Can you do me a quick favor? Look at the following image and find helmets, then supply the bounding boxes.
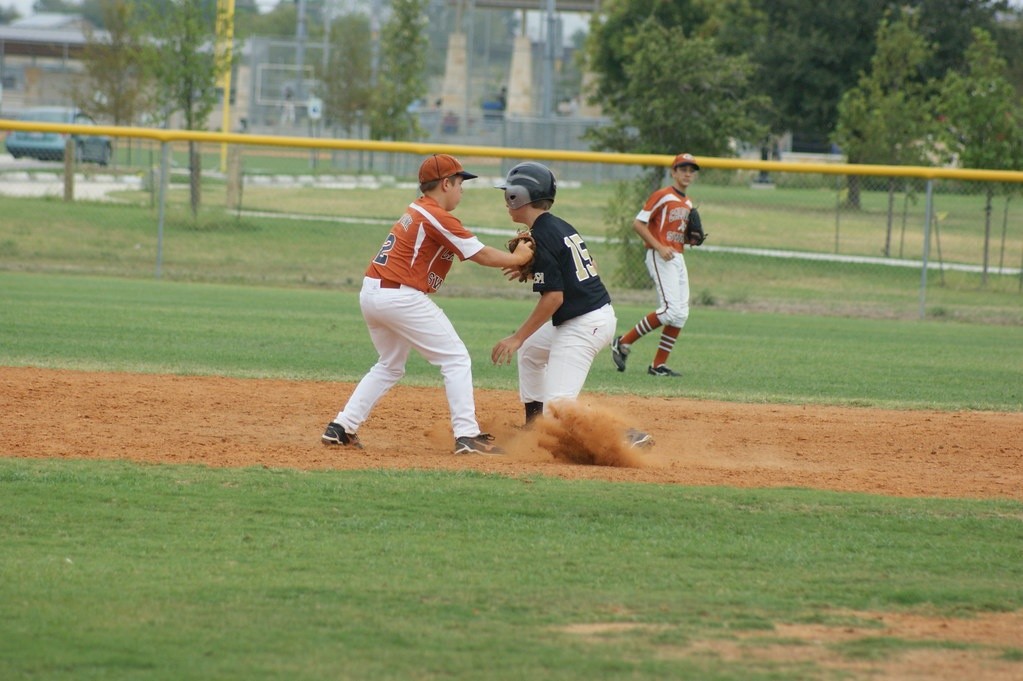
[494,162,557,210]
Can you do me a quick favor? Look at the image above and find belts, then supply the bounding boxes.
[380,279,400,289]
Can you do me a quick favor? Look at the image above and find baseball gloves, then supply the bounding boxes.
[684,207,704,246]
[504,226,536,283]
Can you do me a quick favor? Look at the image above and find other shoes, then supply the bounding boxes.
[626,428,655,450]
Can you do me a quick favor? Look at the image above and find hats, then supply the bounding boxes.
[419,153,478,183]
[673,153,700,171]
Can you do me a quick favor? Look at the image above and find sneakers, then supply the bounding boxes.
[455,434,506,456]
[648,363,682,377]
[323,422,364,449]
[611,335,631,372]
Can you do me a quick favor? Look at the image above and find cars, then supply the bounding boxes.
[7,108,112,166]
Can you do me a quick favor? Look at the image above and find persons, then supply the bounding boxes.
[492,160,656,454]
[610,154,700,376]
[321,154,534,458]
